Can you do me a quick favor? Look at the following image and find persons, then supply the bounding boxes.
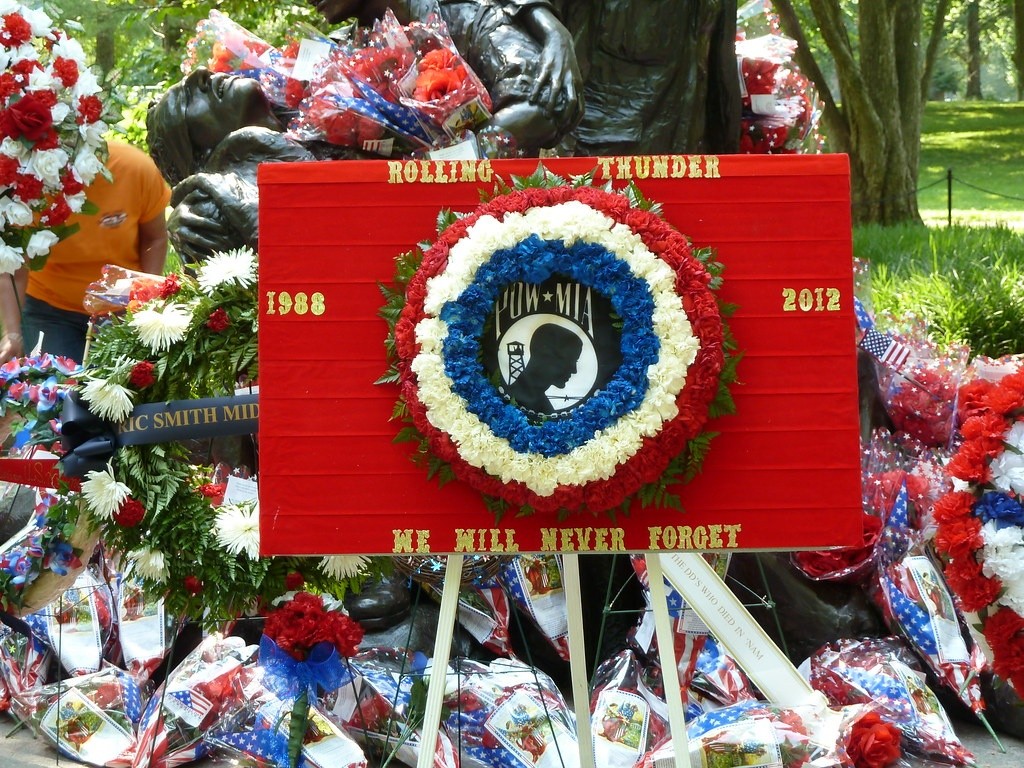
[0,141,170,368]
[144,2,752,282]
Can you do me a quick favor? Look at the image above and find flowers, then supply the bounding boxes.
[373,158,749,524]
[0,244,1024,768]
[734,0,830,159]
[0,0,125,278]
[168,0,527,160]
